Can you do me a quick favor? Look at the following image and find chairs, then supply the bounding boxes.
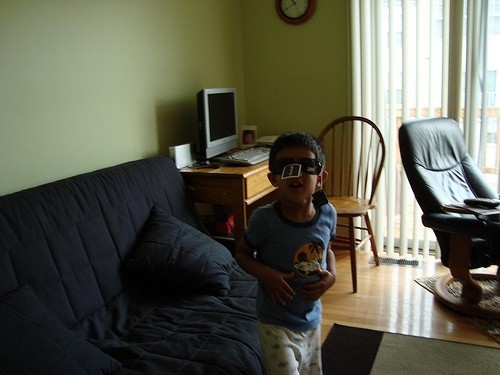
[398,117,500,318]
[315,116,385,294]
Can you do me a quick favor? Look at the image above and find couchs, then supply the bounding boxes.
[0,156,262,375]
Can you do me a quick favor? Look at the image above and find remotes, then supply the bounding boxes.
[464,198,500,209]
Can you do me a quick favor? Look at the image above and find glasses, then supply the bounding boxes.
[270,156,323,174]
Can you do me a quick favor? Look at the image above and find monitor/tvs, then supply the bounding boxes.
[197,87,239,158]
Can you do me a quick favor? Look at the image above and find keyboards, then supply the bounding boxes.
[220,146,271,164]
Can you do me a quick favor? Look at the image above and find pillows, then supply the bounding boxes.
[0,281,124,375]
[117,206,233,298]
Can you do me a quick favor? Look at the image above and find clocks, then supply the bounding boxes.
[274,0,318,26]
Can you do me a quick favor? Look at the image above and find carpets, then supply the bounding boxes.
[321,323,500,375]
[413,272,500,342]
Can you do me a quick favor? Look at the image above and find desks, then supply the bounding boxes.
[178,145,278,252]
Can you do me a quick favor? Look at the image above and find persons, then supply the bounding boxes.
[236,131,337,375]
